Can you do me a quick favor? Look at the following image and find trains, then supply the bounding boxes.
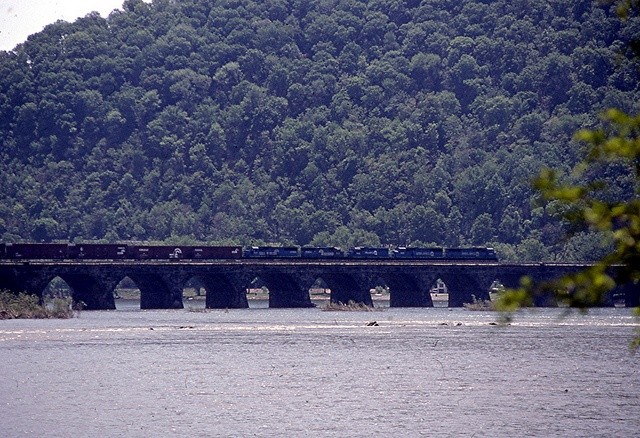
[0,243,503,261]
